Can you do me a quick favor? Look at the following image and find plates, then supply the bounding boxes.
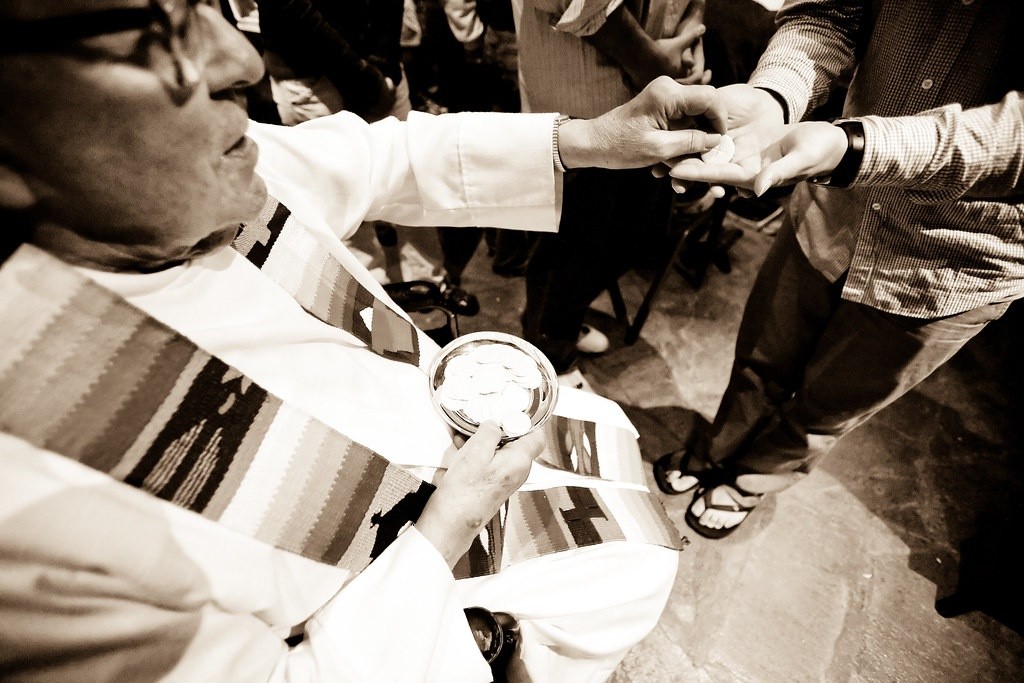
[427,330,560,445]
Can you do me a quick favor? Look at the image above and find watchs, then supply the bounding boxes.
[551,113,571,173]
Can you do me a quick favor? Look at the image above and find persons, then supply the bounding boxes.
[1,0,730,683]
[215,0,1024,541]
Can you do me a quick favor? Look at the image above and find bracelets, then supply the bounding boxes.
[814,121,864,187]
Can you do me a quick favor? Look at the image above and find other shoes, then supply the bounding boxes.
[575,324,610,353]
[556,369,594,394]
[436,283,481,318]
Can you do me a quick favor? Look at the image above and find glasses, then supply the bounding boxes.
[41,0,208,89]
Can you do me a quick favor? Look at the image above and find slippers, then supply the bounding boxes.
[685,484,758,540]
[654,448,708,495]
